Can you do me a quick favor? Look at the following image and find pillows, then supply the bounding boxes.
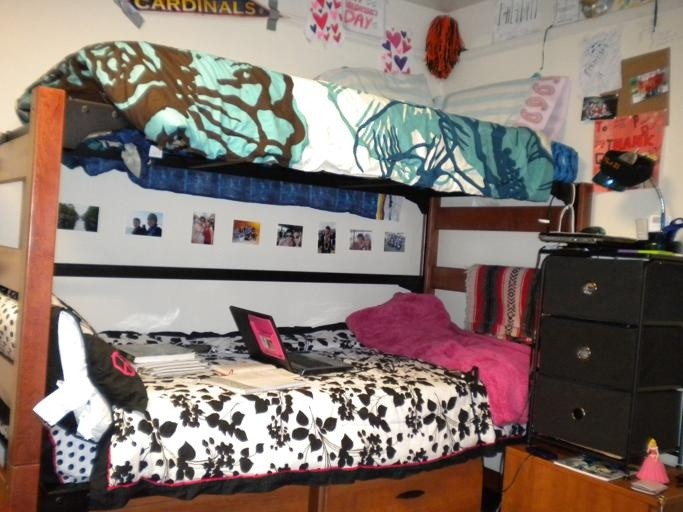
[315,67,567,131]
[0,290,149,446]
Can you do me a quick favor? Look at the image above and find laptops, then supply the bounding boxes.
[227,304,353,375]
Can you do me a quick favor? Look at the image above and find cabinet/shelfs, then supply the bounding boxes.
[497,254,681,511]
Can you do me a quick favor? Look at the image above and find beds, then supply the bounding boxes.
[0,41,593,512]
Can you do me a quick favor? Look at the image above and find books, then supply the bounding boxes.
[206,359,307,395]
[113,343,208,377]
[553,455,639,482]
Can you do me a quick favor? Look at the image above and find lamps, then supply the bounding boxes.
[591,149,673,244]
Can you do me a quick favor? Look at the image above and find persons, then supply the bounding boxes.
[320,225,334,252]
[278,229,301,247]
[636,438,671,484]
[636,68,665,99]
[357,233,370,250]
[583,101,608,118]
[192,216,213,244]
[131,213,161,237]
